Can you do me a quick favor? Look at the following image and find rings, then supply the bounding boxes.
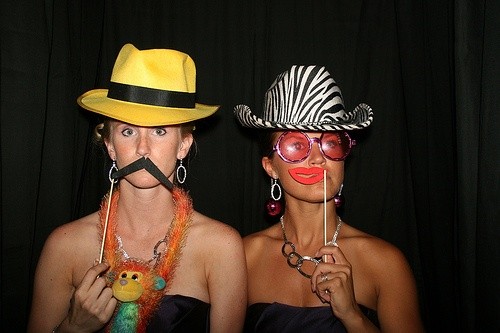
[321,273,328,282]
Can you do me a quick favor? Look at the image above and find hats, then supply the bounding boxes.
[78,45,220,126]
[233,65,374,129]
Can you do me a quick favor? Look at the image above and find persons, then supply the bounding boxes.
[235,65,421,333]
[30,43,248,333]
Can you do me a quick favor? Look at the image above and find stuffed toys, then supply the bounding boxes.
[98,187,193,332]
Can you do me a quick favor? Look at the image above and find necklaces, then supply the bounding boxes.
[280,215,342,278]
[114,231,169,270]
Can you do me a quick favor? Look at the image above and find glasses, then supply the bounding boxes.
[267,131,357,162]
